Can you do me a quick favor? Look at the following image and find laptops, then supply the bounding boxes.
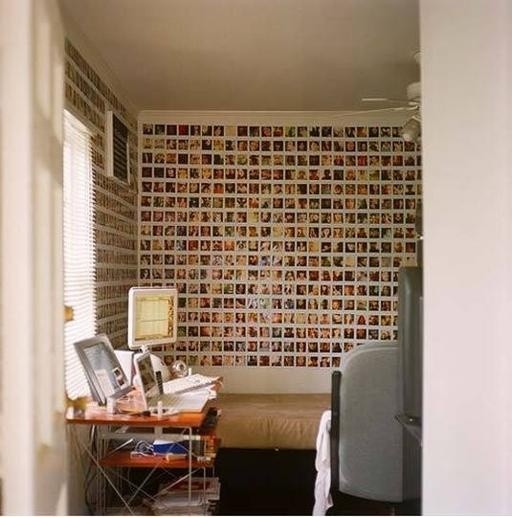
[134,352,211,412]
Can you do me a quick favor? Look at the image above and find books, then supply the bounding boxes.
[145,373,223,412]
[151,477,221,516]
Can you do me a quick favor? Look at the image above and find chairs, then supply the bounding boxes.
[330,343,406,516]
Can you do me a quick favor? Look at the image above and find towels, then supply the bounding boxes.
[312,411,334,516]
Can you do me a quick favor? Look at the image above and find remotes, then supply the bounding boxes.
[155,371,163,394]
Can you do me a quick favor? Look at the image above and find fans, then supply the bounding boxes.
[347,80,421,115]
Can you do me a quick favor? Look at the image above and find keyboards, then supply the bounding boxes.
[161,373,216,395]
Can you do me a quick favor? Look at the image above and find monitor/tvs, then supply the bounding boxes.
[127,287,178,348]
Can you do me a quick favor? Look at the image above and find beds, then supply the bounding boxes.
[111,353,333,450]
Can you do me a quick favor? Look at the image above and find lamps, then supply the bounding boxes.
[399,118,422,143]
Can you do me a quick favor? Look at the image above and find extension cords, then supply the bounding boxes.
[130,451,141,460]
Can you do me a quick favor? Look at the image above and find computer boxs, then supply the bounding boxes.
[113,407,221,505]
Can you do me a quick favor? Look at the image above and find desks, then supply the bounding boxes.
[66,373,218,517]
